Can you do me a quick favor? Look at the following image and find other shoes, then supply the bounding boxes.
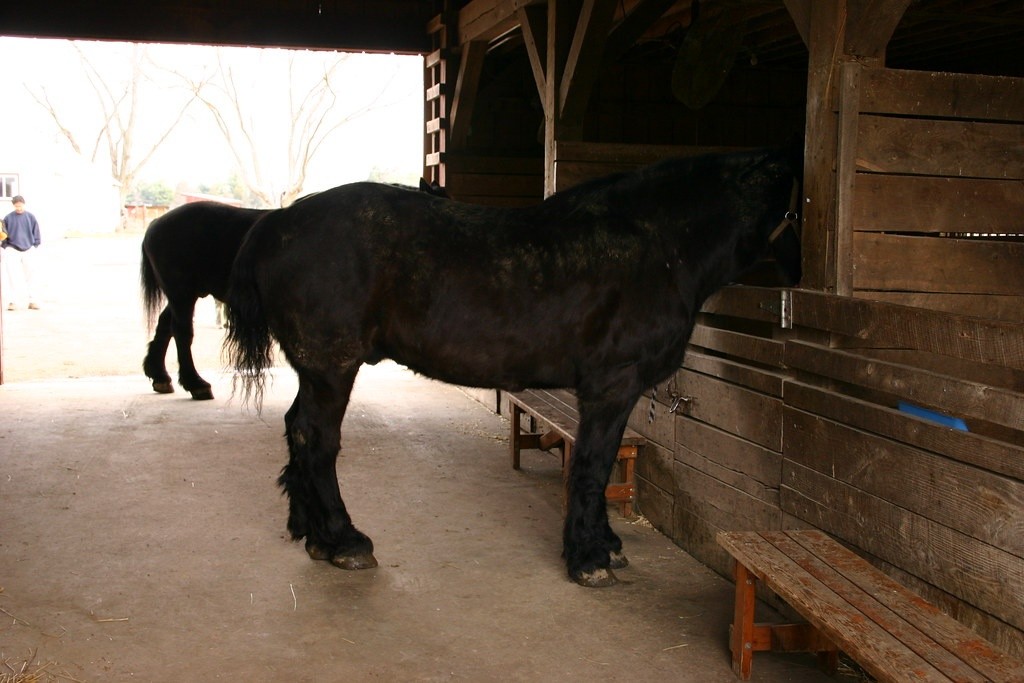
[29,303,39,310]
[8,303,15,311]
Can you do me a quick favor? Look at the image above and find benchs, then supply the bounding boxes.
[502,386,645,519]
[711,528,1023,683]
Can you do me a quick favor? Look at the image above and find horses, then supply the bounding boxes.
[225,144,803,588]
[142,202,277,401]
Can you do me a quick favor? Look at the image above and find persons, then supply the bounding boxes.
[1,195,45,311]
